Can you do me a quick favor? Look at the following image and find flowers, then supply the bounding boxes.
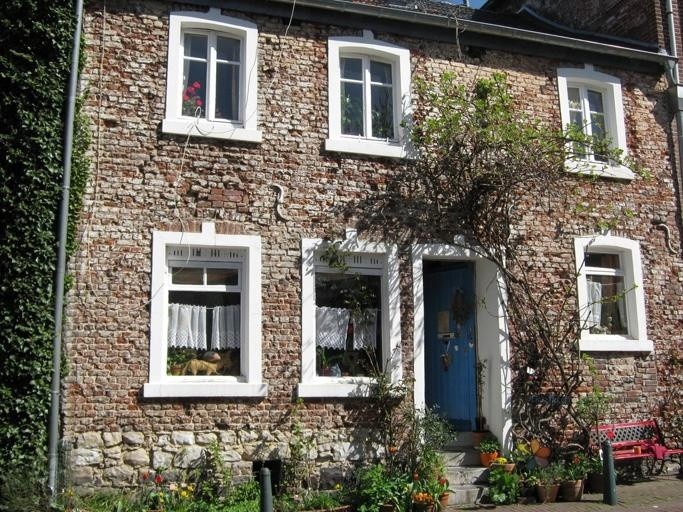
[183,80,205,117]
[403,468,456,512]
[495,456,507,465]
[567,450,591,479]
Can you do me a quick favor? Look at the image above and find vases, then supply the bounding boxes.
[561,478,588,502]
[491,462,515,474]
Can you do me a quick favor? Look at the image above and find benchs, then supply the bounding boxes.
[585,417,681,485]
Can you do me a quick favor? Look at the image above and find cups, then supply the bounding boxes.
[633,445,642,455]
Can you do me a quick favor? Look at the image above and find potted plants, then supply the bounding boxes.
[477,440,501,467]
[315,344,342,375]
[167,348,197,376]
[376,477,402,512]
[524,463,565,502]
